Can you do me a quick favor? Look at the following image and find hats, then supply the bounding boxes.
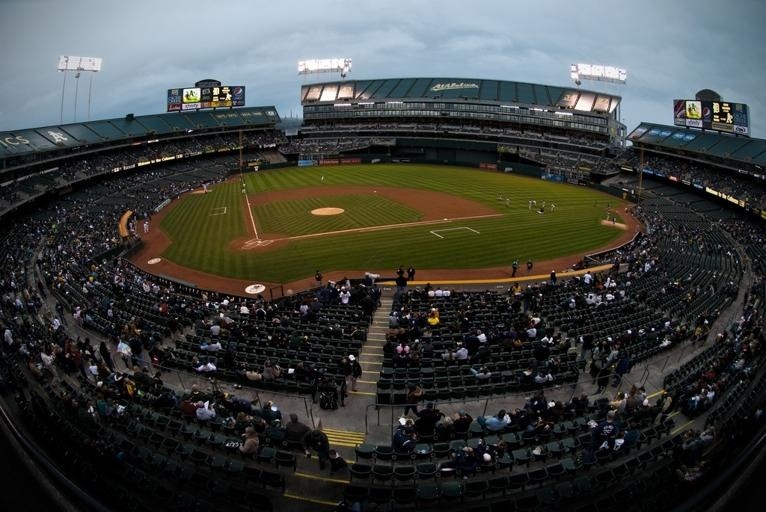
[348,354,356,361]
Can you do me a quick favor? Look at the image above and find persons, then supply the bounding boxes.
[494,191,560,216]
[621,145,765,512]
[1,120,241,512]
[242,244,621,511]
[604,203,617,226]
[242,124,621,185]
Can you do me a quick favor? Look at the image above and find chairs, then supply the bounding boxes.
[660,300,766,512]
[297,114,619,188]
[1,125,296,376]
[372,286,583,410]
[338,394,706,511]
[1,252,381,511]
[149,274,390,411]
[597,139,764,327]
[521,273,693,370]
